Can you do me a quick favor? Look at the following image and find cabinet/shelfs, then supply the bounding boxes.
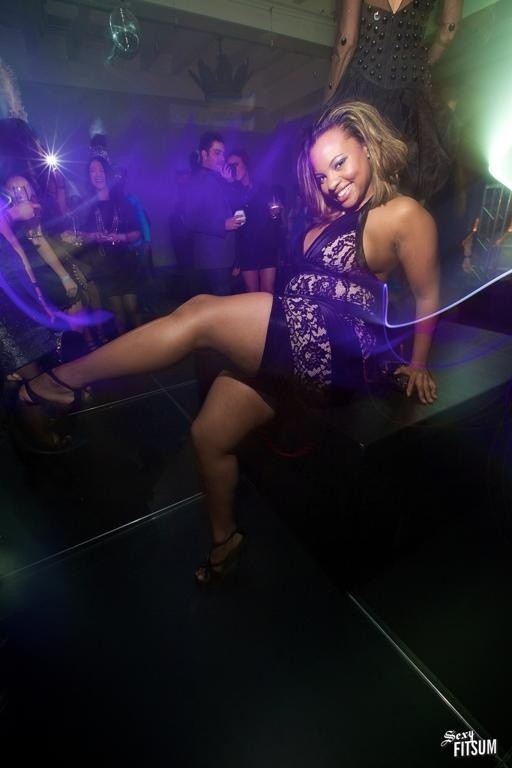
[191,322,512,598]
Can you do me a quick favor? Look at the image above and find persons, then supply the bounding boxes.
[7,102,441,581]
[320,0,464,112]
[1,119,309,378]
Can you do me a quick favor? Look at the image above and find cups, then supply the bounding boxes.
[234,210,245,225]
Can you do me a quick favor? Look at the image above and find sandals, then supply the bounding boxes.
[193,527,253,587]
[85,333,107,351]
[1,369,76,410]
